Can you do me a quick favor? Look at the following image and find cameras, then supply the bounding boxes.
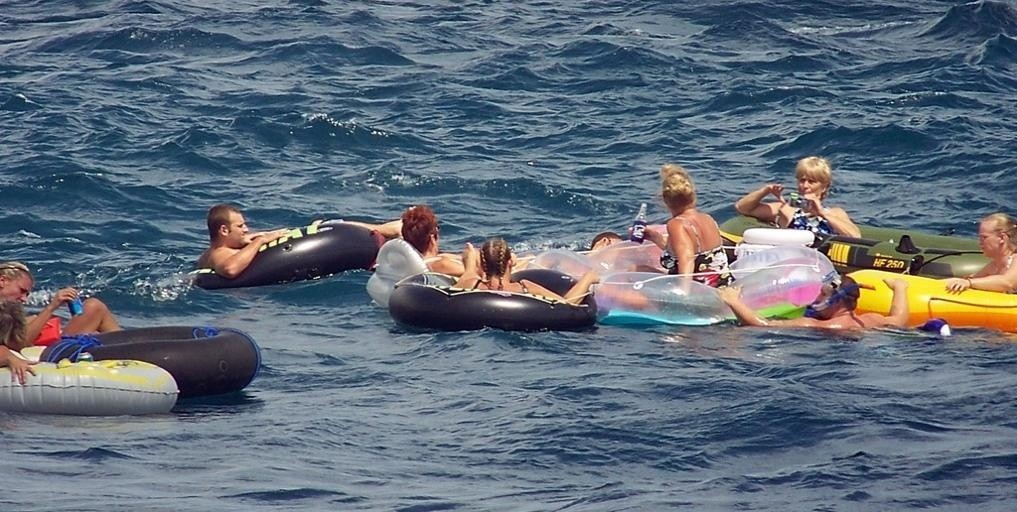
[788,193,809,208]
[65,291,90,315]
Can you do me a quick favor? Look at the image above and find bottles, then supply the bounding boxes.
[629,204,649,243]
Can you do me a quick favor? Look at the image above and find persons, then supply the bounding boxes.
[630,161,731,289]
[946,213,1017,293]
[1,259,118,339]
[199,205,419,278]
[402,204,537,275]
[0,300,36,385]
[717,271,910,329]
[591,232,626,253]
[735,156,862,239]
[451,237,601,305]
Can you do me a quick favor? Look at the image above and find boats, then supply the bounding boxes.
[719,213,1016,332]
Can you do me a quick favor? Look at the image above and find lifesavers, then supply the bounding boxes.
[389,268,598,333]
[845,269,1017,333]
[365,238,429,309]
[35,325,262,399]
[0,346,180,416]
[194,223,379,290]
[526,239,836,326]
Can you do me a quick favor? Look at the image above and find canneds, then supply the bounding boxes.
[75,352,93,363]
[67,298,85,318]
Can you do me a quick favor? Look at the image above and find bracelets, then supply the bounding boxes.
[968,278,975,289]
